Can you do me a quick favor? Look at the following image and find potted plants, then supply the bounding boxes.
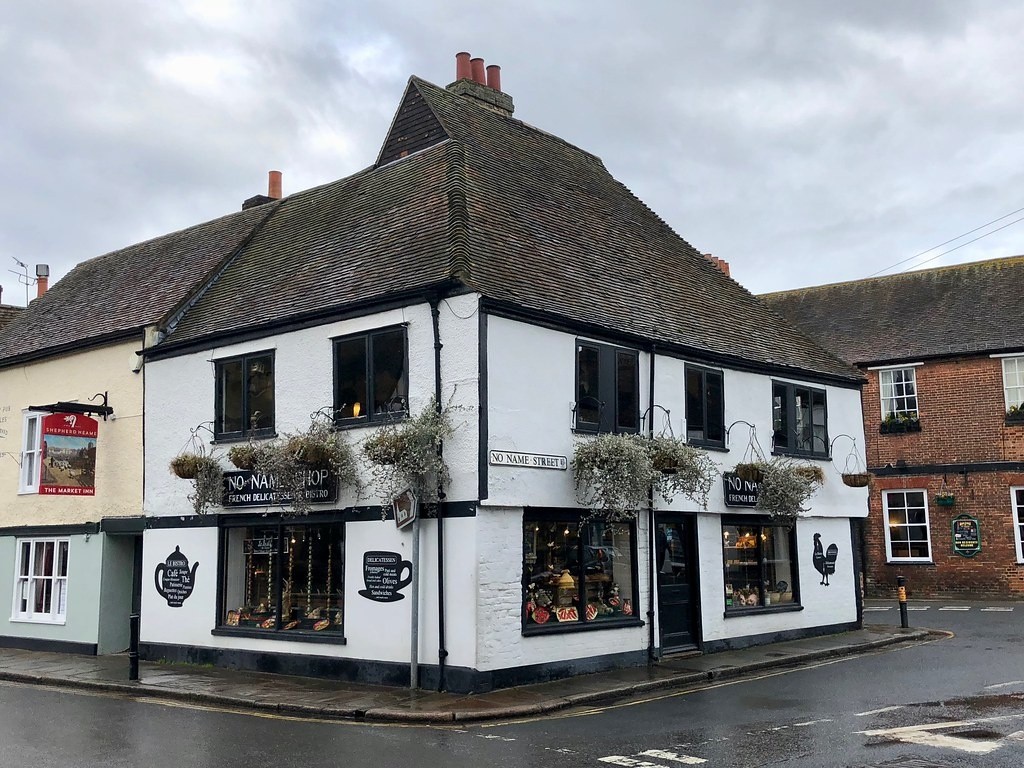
[933,492,957,507]
[226,384,875,523]
[168,444,230,515]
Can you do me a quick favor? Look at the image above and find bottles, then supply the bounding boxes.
[726,594,733,607]
[765,594,770,604]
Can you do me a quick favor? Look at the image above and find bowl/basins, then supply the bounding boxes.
[783,591,792,602]
[770,593,780,604]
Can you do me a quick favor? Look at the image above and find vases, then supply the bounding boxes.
[1007,414,1015,420]
[1014,413,1024,420]
[882,421,919,431]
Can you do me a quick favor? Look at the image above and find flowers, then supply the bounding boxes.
[881,405,1024,429]
